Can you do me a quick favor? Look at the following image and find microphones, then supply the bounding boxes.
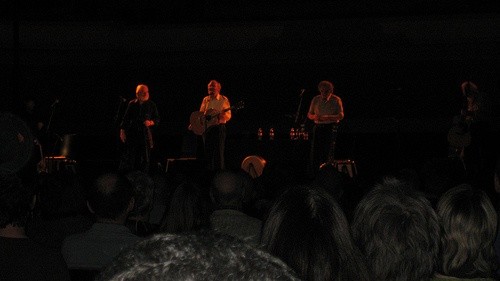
[299,89,305,96]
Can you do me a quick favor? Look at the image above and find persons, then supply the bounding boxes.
[456,82,482,163]
[189,80,232,171]
[307,81,344,156]
[120,83,160,168]
[0,155,500,281]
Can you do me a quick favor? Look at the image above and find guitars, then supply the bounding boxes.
[188,103,244,135]
[35,140,52,175]
[445,102,478,153]
